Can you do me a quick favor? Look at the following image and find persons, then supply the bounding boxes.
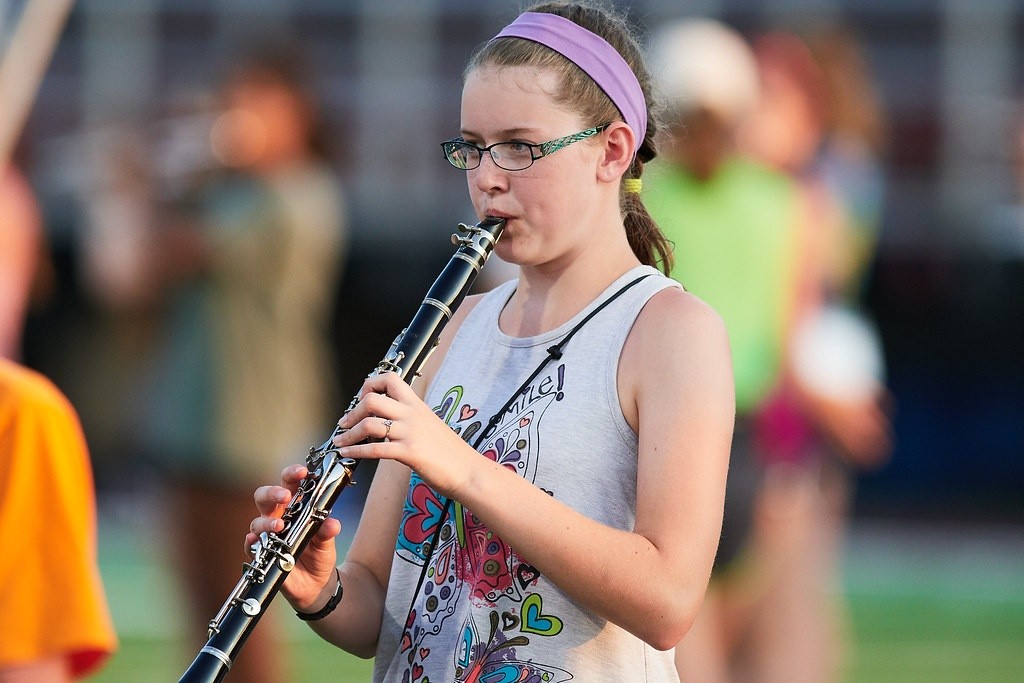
[627,18,896,679]
[0,161,120,681]
[242,3,737,683]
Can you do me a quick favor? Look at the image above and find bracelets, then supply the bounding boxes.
[295,567,344,622]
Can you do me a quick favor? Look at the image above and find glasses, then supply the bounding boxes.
[440,121,613,171]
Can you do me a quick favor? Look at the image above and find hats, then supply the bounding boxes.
[643,17,756,124]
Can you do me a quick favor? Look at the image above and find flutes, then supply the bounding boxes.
[175,211,513,683]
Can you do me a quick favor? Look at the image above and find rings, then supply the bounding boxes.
[382,419,393,440]
